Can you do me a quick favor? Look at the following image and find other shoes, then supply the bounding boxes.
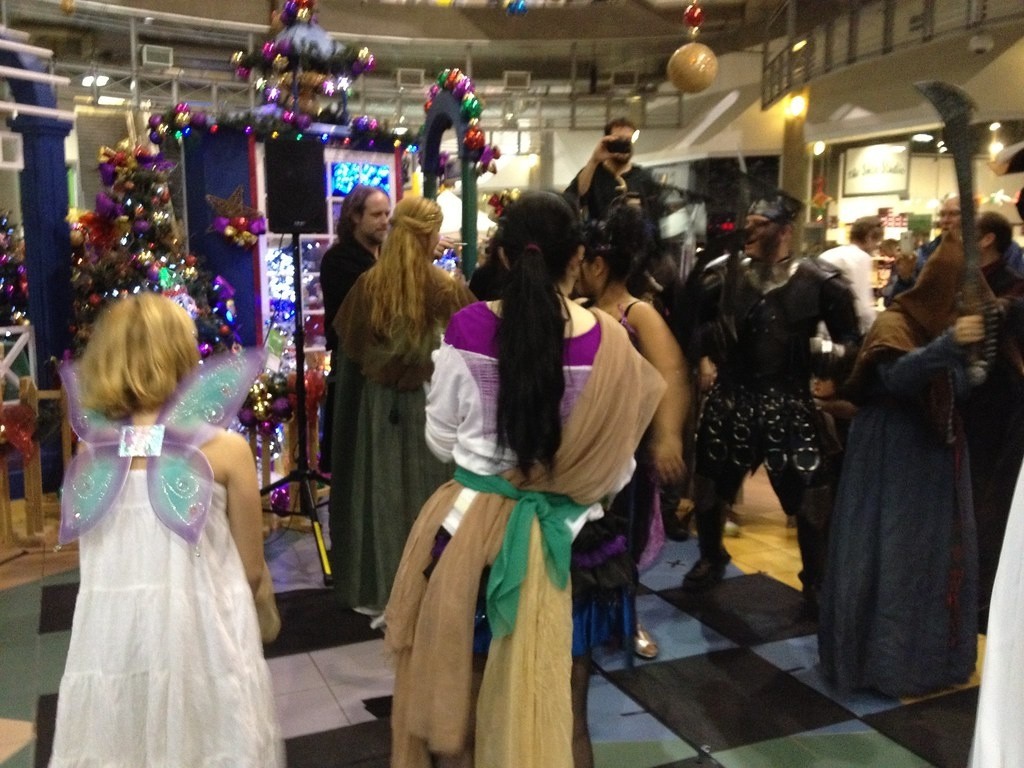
[633,624,657,657]
[684,547,730,591]
[802,585,819,604]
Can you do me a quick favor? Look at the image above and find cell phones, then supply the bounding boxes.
[606,139,634,154]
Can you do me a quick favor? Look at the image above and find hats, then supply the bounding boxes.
[748,198,792,226]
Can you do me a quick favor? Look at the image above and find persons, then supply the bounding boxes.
[683,190,864,622]
[567,218,690,663]
[48,292,287,768]
[817,238,1000,698]
[629,184,1024,498]
[562,118,660,225]
[322,182,393,587]
[384,189,666,768]
[336,191,474,625]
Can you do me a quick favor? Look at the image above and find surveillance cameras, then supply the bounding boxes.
[967,29,993,56]
